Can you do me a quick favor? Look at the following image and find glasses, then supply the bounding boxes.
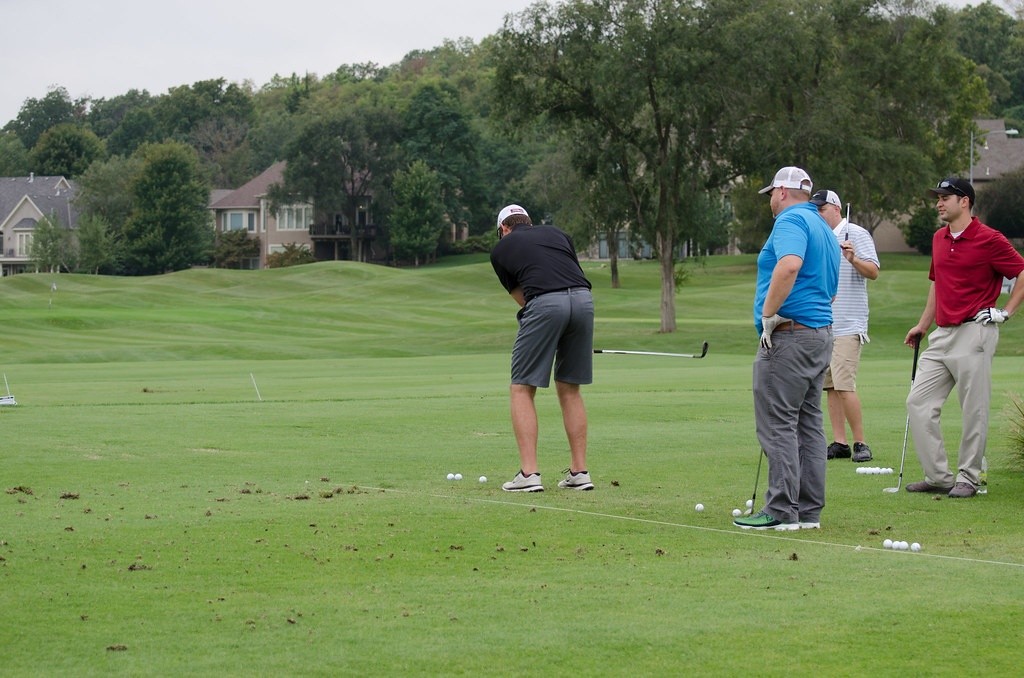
[935,180,967,195]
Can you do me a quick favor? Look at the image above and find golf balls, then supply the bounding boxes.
[447,473,454,480]
[856,467,893,474]
[695,504,704,512]
[746,500,752,508]
[455,474,462,480]
[911,543,920,551]
[732,508,741,517]
[479,476,487,483]
[899,541,908,550]
[892,541,900,549]
[883,539,892,548]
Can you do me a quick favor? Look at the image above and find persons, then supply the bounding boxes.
[810,190,880,463]
[903,178,1024,497]
[490,205,594,494]
[733,167,841,529]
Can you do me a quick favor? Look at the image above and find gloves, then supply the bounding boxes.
[759,313,792,349]
[972,307,1006,327]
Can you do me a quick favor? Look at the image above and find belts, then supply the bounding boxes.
[774,320,813,331]
[542,285,592,293]
[961,318,976,323]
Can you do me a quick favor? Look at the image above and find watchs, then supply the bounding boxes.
[998,307,1009,322]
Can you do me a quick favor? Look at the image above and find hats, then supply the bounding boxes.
[925,176,978,204]
[496,204,533,237]
[758,164,815,195]
[810,189,844,210]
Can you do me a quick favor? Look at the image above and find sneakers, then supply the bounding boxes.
[797,518,821,529]
[556,467,595,490]
[500,469,544,492]
[731,510,800,532]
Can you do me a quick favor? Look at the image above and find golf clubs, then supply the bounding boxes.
[882,333,922,493]
[743,448,763,517]
[591,341,708,358]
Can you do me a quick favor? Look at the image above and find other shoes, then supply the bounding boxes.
[851,442,874,462]
[824,440,852,460]
[906,478,955,495]
[948,480,976,499]
